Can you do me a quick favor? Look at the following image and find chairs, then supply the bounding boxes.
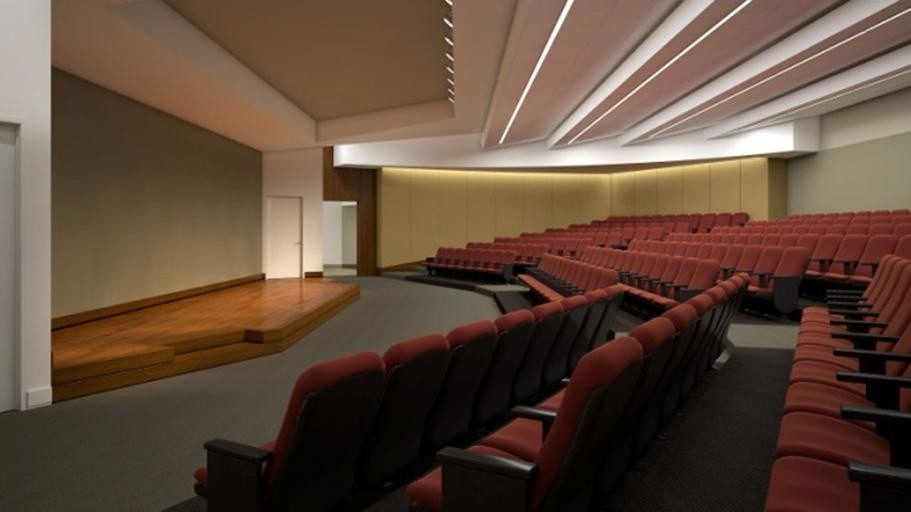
[197,255,911,510]
[422,213,910,316]
